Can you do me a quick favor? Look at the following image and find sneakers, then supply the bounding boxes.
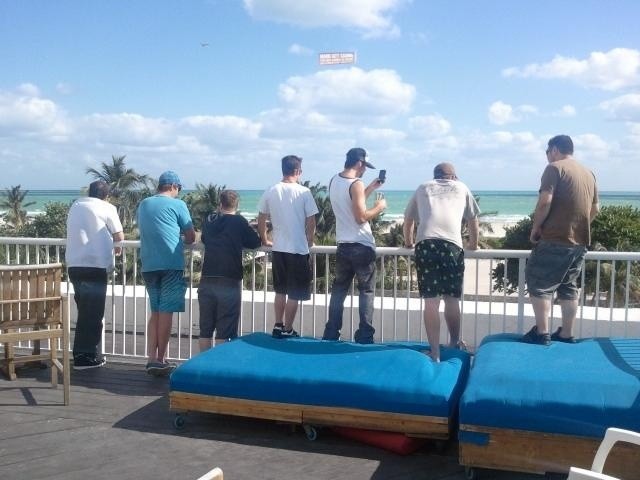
[272,323,299,339]
[73,356,107,369]
[551,326,576,343]
[518,325,551,346]
[146,360,176,376]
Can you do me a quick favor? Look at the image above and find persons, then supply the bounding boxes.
[257,155,318,339]
[136,170,197,375]
[197,190,261,352]
[321,146,389,343]
[65,181,124,371]
[403,161,480,363]
[518,135,599,345]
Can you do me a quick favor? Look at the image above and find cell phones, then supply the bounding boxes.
[379,170,386,183]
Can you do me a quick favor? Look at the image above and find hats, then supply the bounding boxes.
[159,171,181,185]
[346,148,376,169]
[434,162,458,179]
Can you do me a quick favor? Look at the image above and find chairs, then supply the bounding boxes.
[563,425,640,480]
[1,261,73,408]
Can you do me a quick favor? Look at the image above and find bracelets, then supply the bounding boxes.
[405,244,413,248]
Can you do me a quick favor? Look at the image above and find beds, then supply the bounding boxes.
[454,332,640,479]
[165,330,472,443]
